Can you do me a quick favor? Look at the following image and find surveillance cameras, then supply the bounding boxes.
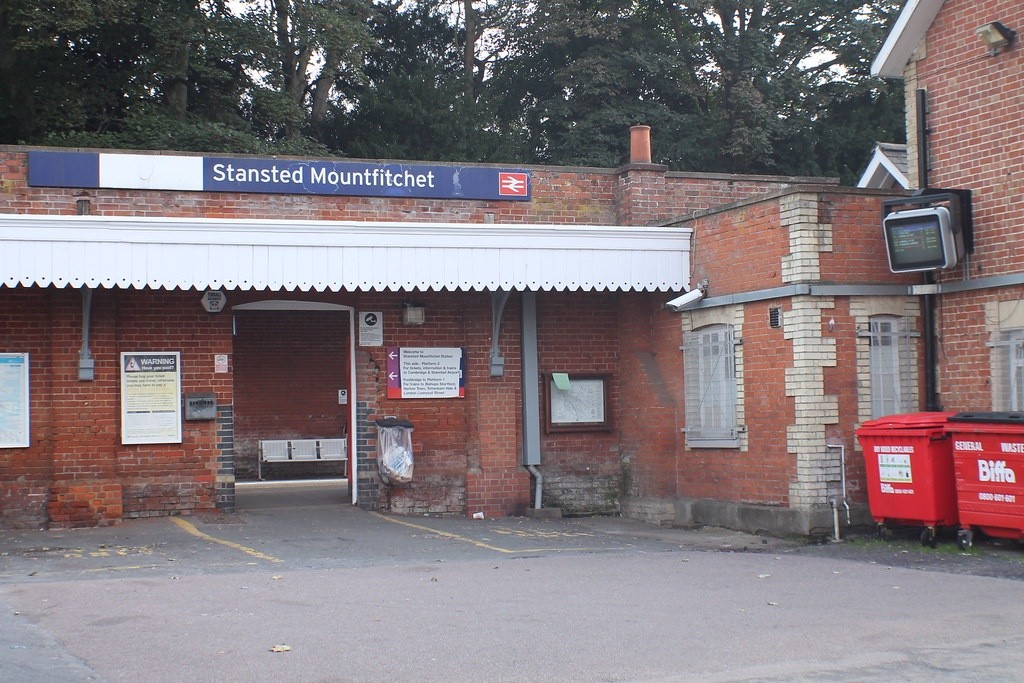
[666,289,703,311]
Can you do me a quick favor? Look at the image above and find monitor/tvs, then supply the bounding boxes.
[883,206,965,274]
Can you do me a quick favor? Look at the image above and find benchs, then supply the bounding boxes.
[256,441,348,479]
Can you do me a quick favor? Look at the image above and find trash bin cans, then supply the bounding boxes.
[944,410,1023,550]
[855,413,960,548]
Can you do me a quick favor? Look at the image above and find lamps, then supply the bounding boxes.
[975,21,1016,48]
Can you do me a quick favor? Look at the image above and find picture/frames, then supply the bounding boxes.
[542,372,612,434]
